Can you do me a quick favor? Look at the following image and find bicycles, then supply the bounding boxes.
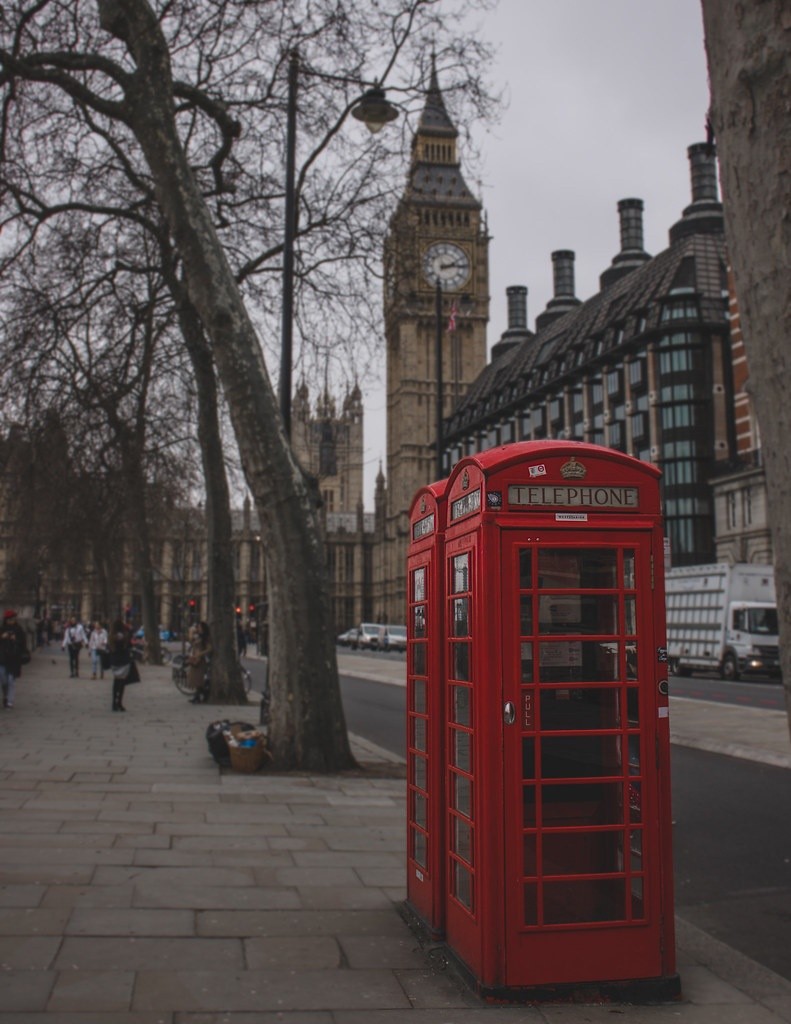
[176,661,252,698]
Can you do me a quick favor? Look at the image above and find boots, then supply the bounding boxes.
[188,680,210,705]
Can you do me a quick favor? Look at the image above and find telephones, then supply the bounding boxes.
[521,620,587,698]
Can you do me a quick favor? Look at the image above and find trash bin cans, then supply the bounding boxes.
[207,718,268,773]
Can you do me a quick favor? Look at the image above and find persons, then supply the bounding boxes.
[0,607,245,713]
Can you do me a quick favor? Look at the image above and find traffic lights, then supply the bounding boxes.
[237,607,242,617]
[249,604,255,613]
[190,597,195,613]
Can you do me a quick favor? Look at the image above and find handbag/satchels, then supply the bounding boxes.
[18,649,31,665]
[96,648,111,670]
[72,642,82,650]
[187,669,205,689]
[112,658,141,686]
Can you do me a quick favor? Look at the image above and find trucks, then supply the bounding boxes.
[664,564,782,680]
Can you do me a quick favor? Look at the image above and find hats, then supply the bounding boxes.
[2,610,18,620]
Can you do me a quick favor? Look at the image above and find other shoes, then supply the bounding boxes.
[113,701,126,711]
[90,672,97,680]
[5,701,13,707]
[100,672,105,680]
[70,670,79,678]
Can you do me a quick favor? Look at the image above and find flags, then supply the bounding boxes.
[446,302,459,333]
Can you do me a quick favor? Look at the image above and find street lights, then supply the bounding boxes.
[280,51,399,443]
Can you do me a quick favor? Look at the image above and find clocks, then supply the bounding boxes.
[420,241,470,290]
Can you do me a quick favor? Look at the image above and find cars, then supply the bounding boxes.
[384,624,408,650]
[359,623,387,649]
